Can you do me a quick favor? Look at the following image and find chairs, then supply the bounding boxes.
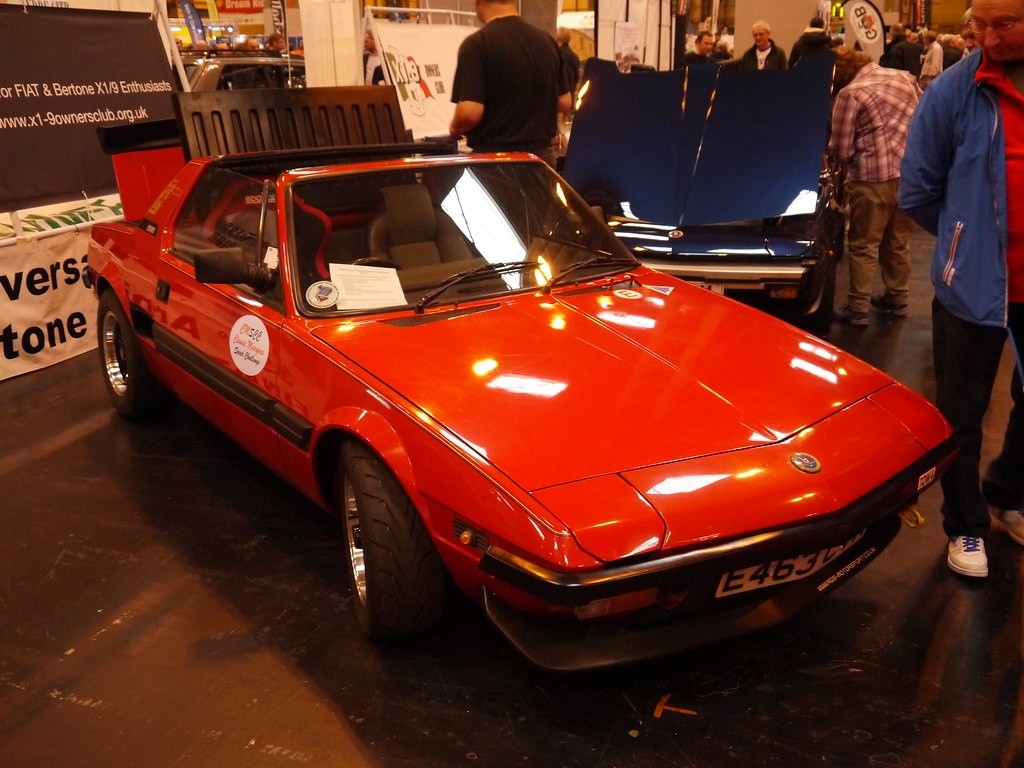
[367,183,475,270]
[199,172,332,283]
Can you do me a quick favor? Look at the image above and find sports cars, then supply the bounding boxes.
[86,134,968,676]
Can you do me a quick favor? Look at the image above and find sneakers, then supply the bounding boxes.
[842,305,870,326]
[988,500,1024,543]
[871,295,909,315]
[947,535,987,576]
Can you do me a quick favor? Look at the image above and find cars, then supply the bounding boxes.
[563,48,846,334]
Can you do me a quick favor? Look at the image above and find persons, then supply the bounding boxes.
[879,23,925,81]
[740,20,787,70]
[363,30,386,86]
[896,0,1024,577]
[192,40,211,58]
[682,31,716,70]
[834,36,844,46]
[714,30,722,46]
[960,7,978,53]
[787,16,836,70]
[232,38,261,89]
[918,30,943,91]
[710,40,731,60]
[828,50,924,325]
[943,34,963,71]
[449,0,572,245]
[905,25,918,46]
[174,37,191,56]
[262,32,289,88]
[556,27,580,111]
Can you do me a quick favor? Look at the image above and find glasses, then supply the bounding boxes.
[968,15,1024,34]
